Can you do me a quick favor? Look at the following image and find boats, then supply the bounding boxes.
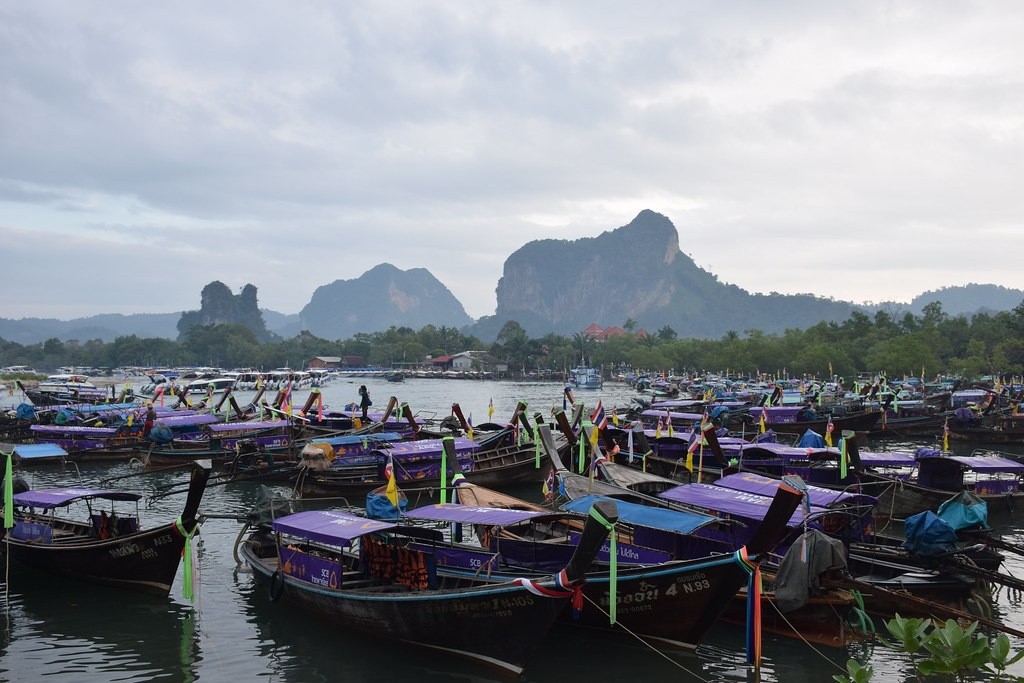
[0,442,213,597]
[0,365,1024,673]
